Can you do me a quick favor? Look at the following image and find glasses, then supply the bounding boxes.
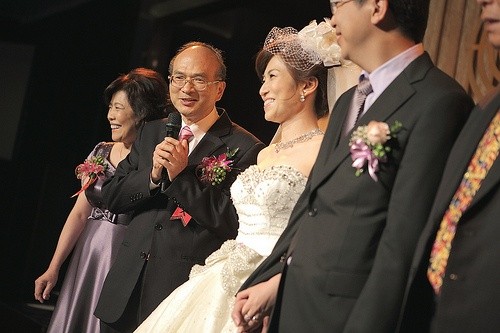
[330,0,348,15]
[167,73,221,90]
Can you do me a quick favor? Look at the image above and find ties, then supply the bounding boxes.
[176,126,195,155]
[425,112,500,293]
[338,76,373,147]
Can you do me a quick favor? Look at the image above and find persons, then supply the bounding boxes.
[84,41,267,333]
[232,0,476,333]
[131,17,367,333]
[394,0,500,333]
[34,67,178,333]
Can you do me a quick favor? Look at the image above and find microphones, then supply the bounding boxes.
[160,112,182,182]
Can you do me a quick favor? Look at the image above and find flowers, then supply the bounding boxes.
[195,145,243,188]
[348,119,405,184]
[298,15,353,68]
[74,155,111,181]
